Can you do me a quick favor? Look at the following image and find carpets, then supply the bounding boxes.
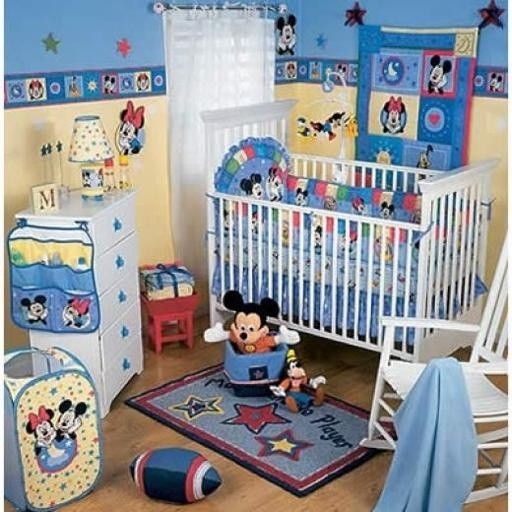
[125,364,395,501]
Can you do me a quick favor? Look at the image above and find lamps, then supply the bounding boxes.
[68,117,117,200]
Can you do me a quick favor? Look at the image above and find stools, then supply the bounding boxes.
[148,311,196,353]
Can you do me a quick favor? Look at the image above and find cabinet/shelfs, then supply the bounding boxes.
[15,187,145,417]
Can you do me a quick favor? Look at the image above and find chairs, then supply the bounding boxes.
[361,234,507,505]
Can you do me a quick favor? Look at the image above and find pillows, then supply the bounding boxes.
[215,136,290,217]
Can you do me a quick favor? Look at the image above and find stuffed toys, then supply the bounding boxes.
[202,291,301,354]
[296,80,358,141]
[270,348,326,412]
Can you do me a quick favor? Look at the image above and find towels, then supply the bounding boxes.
[374,347,480,511]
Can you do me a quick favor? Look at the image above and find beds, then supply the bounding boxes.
[199,104,499,366]
